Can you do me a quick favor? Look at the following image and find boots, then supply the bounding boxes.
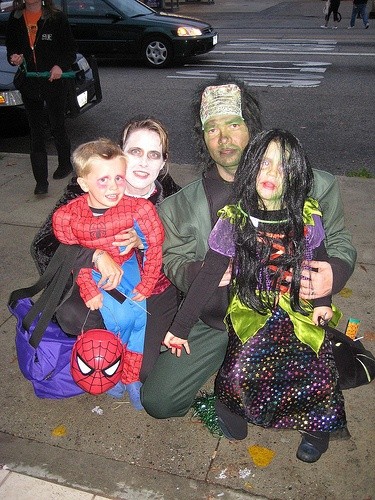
[30,149,50,194]
[52,138,73,179]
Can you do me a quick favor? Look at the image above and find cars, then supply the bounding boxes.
[0,0,219,70]
[0,44,104,138]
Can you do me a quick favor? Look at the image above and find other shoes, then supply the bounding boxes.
[211,396,250,440]
[364,23,369,29]
[332,26,338,30]
[348,26,355,30]
[296,437,329,464]
[320,25,327,28]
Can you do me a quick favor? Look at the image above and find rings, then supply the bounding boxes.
[310,289,314,295]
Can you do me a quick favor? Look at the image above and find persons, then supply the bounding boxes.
[321,0,341,29]
[5,0,78,195]
[349,0,370,29]
[31,73,357,464]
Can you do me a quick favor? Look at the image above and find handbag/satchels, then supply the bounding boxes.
[13,63,27,91]
[319,317,375,388]
[323,0,330,15]
[7,242,87,399]
